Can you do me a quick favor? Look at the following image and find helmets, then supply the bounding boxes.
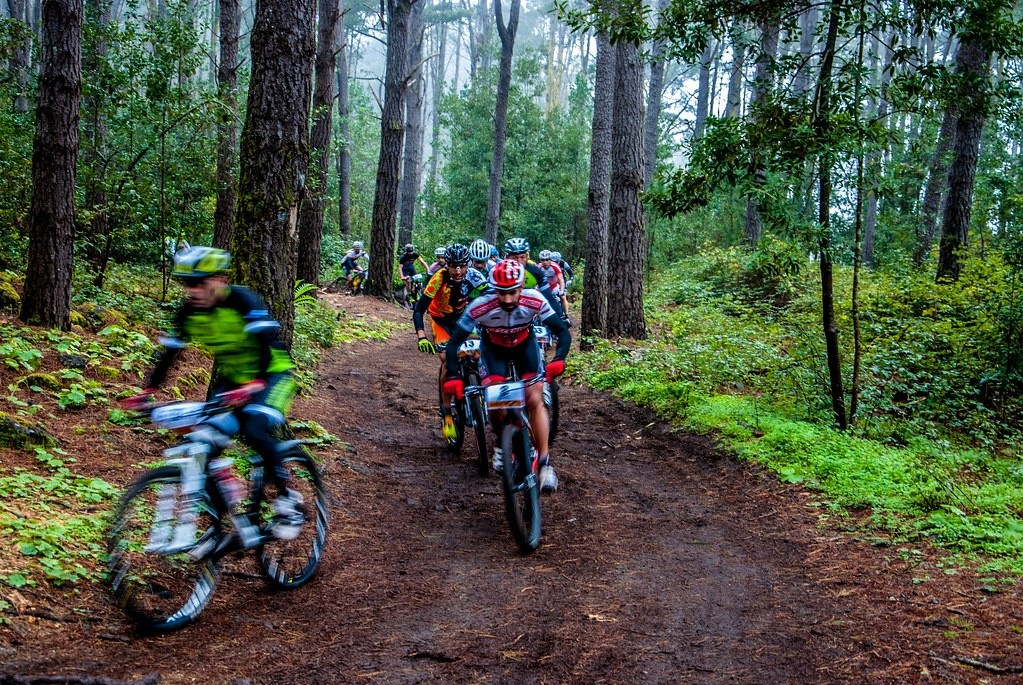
[434,247,446,256]
[444,244,470,262]
[170,247,231,274]
[552,252,562,261]
[488,259,524,291]
[354,241,363,250]
[405,244,414,251]
[505,238,530,253]
[539,250,552,258]
[490,245,499,256]
[469,239,491,262]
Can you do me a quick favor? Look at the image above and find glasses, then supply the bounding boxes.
[495,285,522,295]
[170,276,217,287]
[447,262,468,268]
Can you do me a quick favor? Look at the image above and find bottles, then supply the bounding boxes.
[209,457,245,504]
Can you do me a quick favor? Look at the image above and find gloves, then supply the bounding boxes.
[545,361,565,382]
[417,337,436,355]
[444,380,463,398]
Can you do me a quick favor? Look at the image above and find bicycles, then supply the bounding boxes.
[104,378,330,640]
[533,279,574,363]
[418,334,493,478]
[400,269,427,310]
[452,366,565,556]
[335,265,369,294]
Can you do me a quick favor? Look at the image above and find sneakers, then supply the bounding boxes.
[492,446,505,472]
[272,491,304,540]
[441,415,459,444]
[538,466,558,493]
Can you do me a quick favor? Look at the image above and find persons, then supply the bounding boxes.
[504,238,571,328]
[398,244,430,309]
[467,238,504,280]
[421,247,447,296]
[412,243,491,444]
[537,249,564,307]
[340,240,369,290]
[550,252,574,324]
[160,228,191,267]
[122,245,306,556]
[442,258,572,495]
